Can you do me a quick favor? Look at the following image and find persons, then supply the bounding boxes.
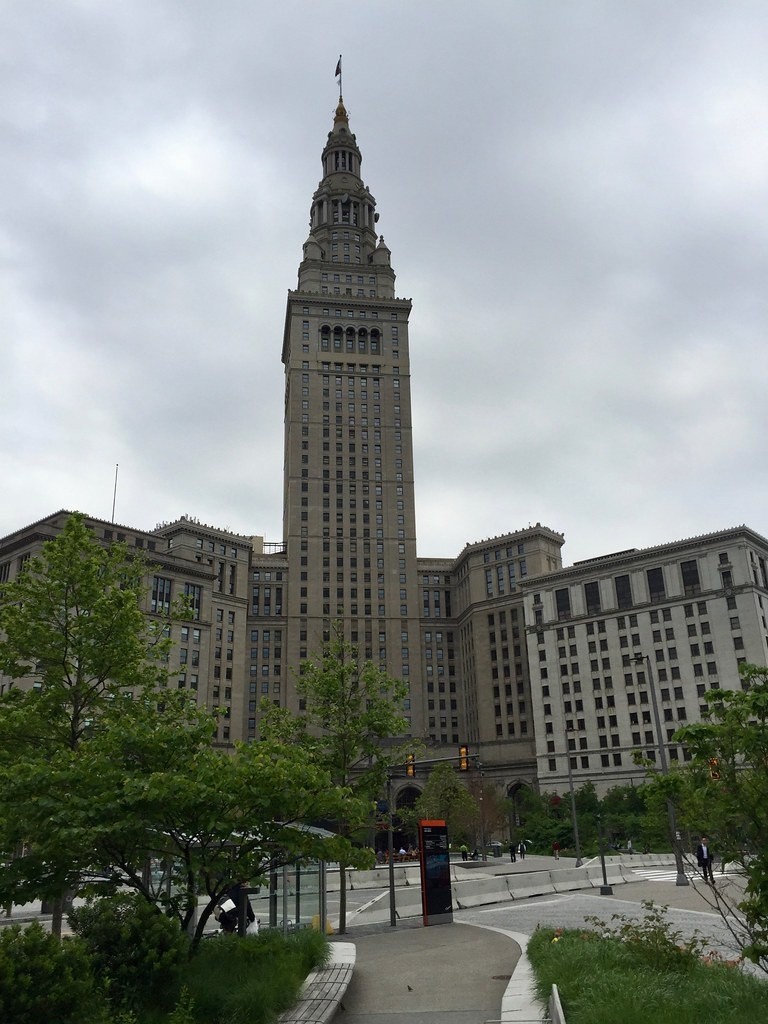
[697,837,715,884]
[628,838,633,855]
[408,843,413,852]
[509,842,516,863]
[399,847,407,861]
[377,848,396,864]
[414,847,419,853]
[493,846,502,857]
[223,881,259,934]
[460,843,467,861]
[467,849,478,860]
[518,840,526,859]
[643,843,650,854]
[608,841,621,850]
[552,840,560,860]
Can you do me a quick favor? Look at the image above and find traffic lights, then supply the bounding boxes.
[709,757,720,780]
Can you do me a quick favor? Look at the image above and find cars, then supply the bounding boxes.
[676,832,700,843]
[486,841,504,847]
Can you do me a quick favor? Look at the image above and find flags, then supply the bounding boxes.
[335,59,340,77]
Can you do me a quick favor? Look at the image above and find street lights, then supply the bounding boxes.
[564,728,584,867]
[626,655,689,886]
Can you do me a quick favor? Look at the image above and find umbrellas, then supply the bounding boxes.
[488,841,504,846]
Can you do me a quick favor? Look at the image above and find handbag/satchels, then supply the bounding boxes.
[246,918,258,936]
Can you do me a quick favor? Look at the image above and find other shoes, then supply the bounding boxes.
[712,881,715,885]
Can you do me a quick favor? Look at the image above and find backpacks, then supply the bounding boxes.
[212,895,238,926]
